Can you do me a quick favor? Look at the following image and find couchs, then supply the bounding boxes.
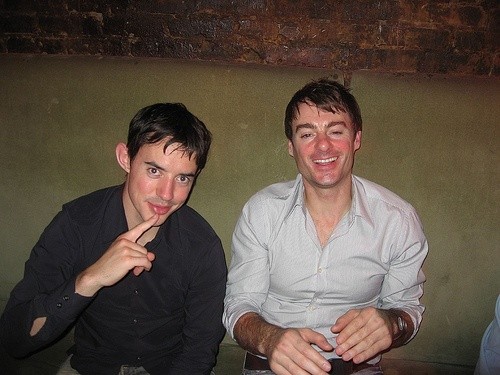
[0,52,500,375]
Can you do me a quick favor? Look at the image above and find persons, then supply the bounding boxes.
[0,103,228,375]
[222,79,429,375]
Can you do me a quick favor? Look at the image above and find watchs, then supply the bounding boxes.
[384,309,408,340]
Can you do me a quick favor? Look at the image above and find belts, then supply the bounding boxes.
[242,351,382,375]
